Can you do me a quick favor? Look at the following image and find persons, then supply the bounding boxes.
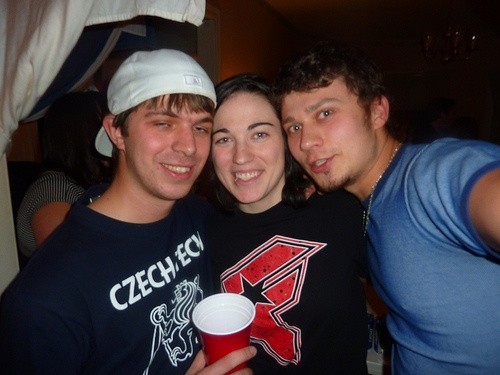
[0,49,257,375]
[280,46,500,374]
[210,72,388,375]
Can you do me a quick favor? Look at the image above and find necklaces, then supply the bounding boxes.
[363,141,403,236]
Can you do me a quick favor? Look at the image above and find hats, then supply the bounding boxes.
[95,49,217,158]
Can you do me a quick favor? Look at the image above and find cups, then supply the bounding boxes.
[192,293,256,375]
